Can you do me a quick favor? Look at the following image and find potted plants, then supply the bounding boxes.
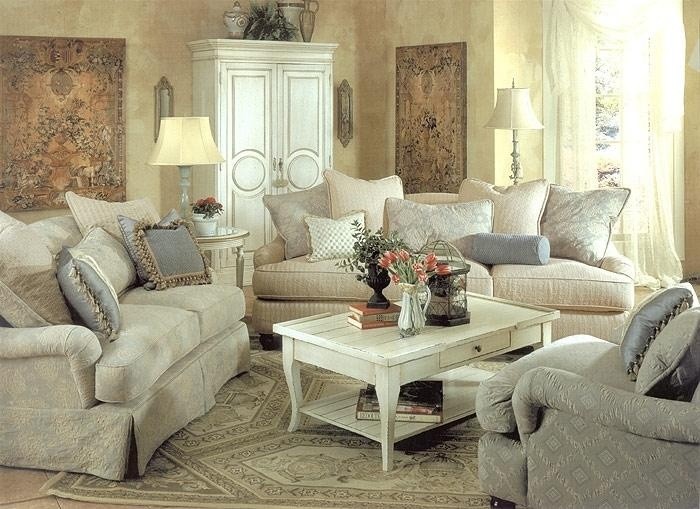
[333,215,412,310]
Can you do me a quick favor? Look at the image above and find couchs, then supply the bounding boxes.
[249,189,642,353]
[0,208,251,482]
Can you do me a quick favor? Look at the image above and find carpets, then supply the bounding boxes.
[37,328,538,509]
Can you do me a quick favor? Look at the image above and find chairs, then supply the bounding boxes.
[475,280,699,509]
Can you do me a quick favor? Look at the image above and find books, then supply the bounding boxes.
[366,386,441,416]
[350,311,402,323]
[355,388,444,425]
[347,315,399,329]
[348,302,402,317]
[364,379,445,410]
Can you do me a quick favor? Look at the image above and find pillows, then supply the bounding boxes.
[620,279,699,382]
[464,229,554,268]
[54,187,213,343]
[321,169,404,240]
[539,183,631,269]
[300,209,368,262]
[457,177,551,237]
[262,182,329,260]
[385,196,495,253]
[634,307,699,404]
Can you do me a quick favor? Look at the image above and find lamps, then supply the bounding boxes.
[482,78,546,184]
[147,115,225,221]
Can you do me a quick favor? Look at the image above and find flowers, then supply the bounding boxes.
[190,197,227,219]
[373,247,454,291]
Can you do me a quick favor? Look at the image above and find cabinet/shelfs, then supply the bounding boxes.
[186,38,342,292]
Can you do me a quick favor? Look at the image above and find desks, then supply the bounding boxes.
[184,220,250,290]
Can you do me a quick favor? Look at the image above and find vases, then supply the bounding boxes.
[192,211,219,236]
[297,0,320,43]
[396,282,432,337]
[277,0,306,42]
[223,1,252,39]
